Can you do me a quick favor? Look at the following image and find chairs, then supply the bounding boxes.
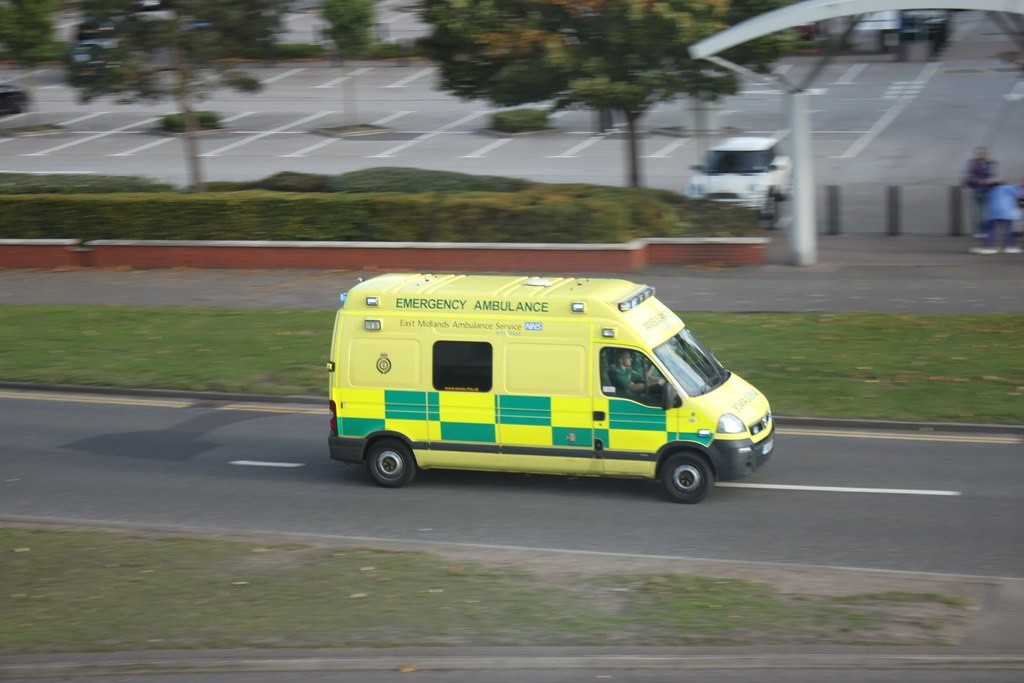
[601,350,616,396]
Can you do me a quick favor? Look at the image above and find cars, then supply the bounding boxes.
[900,9,950,50]
[72,38,124,84]
[686,137,792,222]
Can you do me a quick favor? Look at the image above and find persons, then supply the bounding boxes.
[969,182,1024,255]
[609,349,666,394]
[963,146,1002,238]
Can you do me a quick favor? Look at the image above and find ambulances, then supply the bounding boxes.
[325,271,775,505]
